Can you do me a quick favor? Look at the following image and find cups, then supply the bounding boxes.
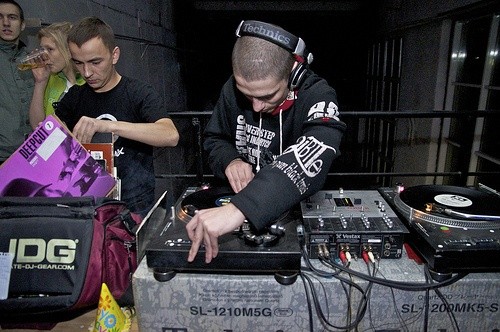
[17,47,50,71]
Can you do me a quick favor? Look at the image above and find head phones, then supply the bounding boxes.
[236,20,315,91]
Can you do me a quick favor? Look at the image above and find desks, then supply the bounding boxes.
[132,243,500,332]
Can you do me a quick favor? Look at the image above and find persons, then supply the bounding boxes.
[26,22,86,129]
[53,16,180,306]
[184,19,347,265]
[0,0,36,166]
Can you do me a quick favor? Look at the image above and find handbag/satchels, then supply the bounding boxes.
[0,197,144,317]
